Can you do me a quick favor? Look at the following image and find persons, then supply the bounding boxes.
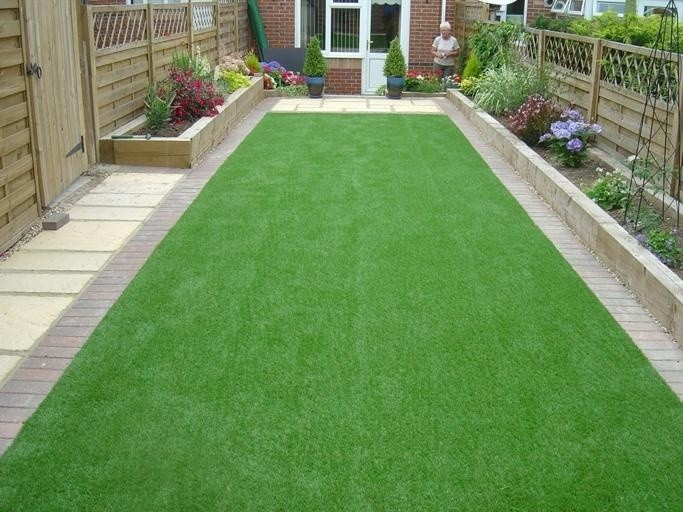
[429,21,461,79]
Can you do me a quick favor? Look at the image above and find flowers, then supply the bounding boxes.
[259,60,304,91]
[509,95,603,167]
[155,69,224,127]
[405,70,461,93]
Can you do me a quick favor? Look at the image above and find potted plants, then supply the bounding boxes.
[382,33,406,99]
[301,34,326,98]
